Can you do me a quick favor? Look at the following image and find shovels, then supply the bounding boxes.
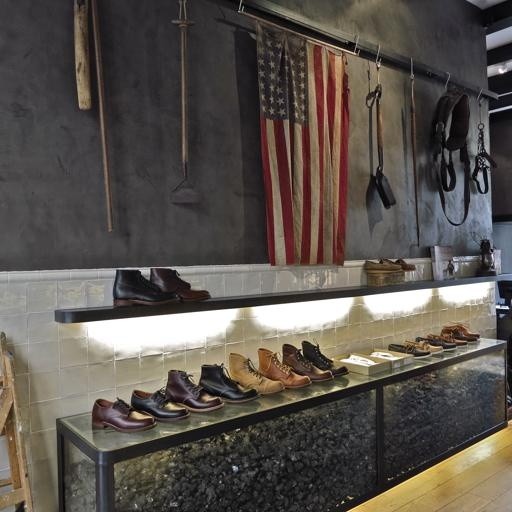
[374,56,396,209]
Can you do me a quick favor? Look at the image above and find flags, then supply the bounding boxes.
[253,22,352,266]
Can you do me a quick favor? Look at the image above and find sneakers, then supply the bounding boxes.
[388,325,480,359]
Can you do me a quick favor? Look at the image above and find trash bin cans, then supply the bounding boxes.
[496,304,511,405]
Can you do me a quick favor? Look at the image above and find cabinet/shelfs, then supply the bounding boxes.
[52,273,512,509]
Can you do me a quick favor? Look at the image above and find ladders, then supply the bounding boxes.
[0,333,33,512]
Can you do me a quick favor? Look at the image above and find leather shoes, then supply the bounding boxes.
[228,340,350,394]
[92,398,157,433]
[200,364,260,404]
[365,258,402,273]
[167,369,225,413]
[151,268,210,302]
[386,257,416,272]
[114,269,180,306]
[131,389,190,422]
